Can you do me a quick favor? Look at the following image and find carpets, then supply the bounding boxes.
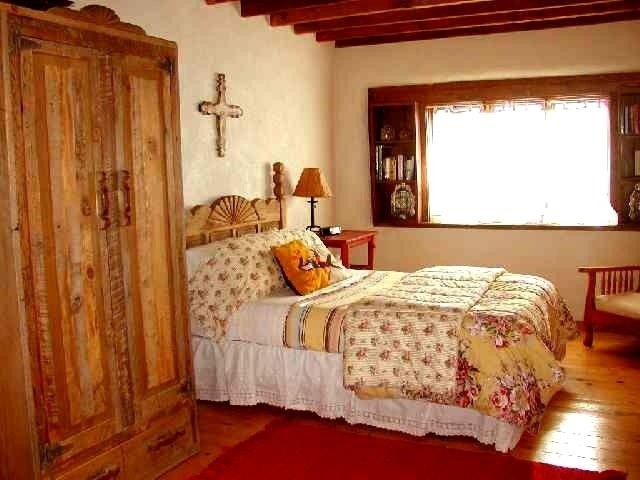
[197,411,630,479]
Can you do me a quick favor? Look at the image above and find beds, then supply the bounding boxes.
[185,162,575,451]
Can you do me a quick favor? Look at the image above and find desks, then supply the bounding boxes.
[315,230,378,270]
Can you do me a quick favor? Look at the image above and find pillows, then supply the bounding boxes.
[265,240,351,293]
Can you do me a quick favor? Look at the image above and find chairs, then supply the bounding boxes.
[576,267,639,347]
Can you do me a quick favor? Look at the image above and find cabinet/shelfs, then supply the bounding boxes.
[368,86,423,228]
[2,5,201,478]
[615,73,640,231]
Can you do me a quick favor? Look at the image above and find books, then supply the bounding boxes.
[375,145,414,181]
[619,101,640,135]
[619,150,640,177]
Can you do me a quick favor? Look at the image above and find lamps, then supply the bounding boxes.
[292,168,332,233]
